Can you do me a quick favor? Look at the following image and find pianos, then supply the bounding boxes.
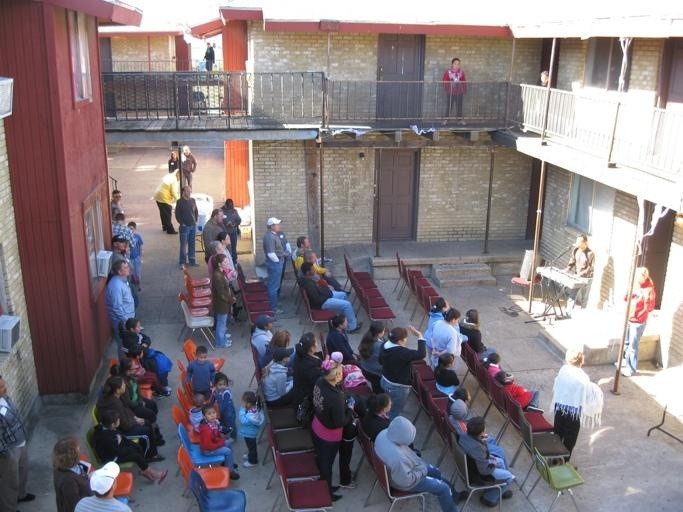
[536,267,587,290]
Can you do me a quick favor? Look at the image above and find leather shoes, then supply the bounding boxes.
[331,486,340,492]
[331,495,343,501]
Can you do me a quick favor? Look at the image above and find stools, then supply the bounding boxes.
[192,233,204,253]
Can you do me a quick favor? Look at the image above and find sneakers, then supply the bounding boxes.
[221,462,238,469]
[225,333,231,338]
[527,405,544,414]
[480,495,498,507]
[340,481,357,489]
[159,434,163,439]
[180,263,184,269]
[18,493,35,502]
[230,471,240,480]
[167,231,178,233]
[162,386,172,391]
[217,340,231,348]
[154,439,165,446]
[243,461,257,467]
[163,229,167,231]
[149,453,166,463]
[459,490,469,500]
[622,368,631,377]
[191,264,200,266]
[347,322,362,334]
[614,359,626,367]
[501,490,513,499]
[242,454,248,459]
[158,391,174,398]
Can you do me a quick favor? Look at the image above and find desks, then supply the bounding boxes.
[643,404,682,444]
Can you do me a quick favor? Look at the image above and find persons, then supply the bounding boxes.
[91,320,170,484]
[262,218,285,315]
[107,190,143,340]
[550,347,591,463]
[493,370,545,413]
[201,42,216,72]
[423,298,461,373]
[373,416,469,511]
[614,266,656,377]
[440,58,467,117]
[72,461,133,512]
[152,168,181,234]
[462,310,499,357]
[293,233,363,333]
[560,234,596,310]
[0,376,36,507]
[237,389,265,470]
[173,186,199,268]
[536,71,551,87]
[482,352,502,378]
[185,346,241,483]
[203,199,244,350]
[423,351,517,508]
[273,229,291,301]
[249,314,426,503]
[51,437,94,511]
[162,139,199,193]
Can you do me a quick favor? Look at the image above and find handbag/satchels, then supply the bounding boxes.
[296,392,316,427]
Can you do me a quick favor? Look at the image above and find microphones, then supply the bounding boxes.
[566,243,577,252]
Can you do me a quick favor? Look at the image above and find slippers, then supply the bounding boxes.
[158,469,169,485]
[139,470,156,481]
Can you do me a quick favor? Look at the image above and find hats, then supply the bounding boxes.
[272,347,294,362]
[111,234,129,242]
[90,461,120,495]
[267,217,281,225]
[256,314,278,325]
